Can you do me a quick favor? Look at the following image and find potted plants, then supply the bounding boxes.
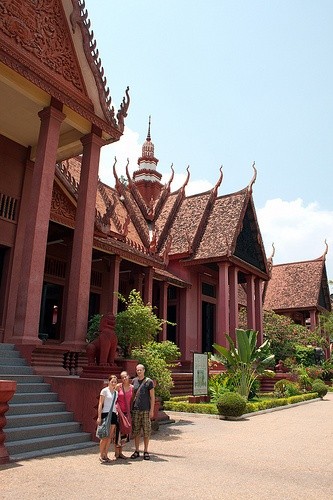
[86,289,177,379]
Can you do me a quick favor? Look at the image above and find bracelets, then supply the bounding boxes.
[98,417,101,419]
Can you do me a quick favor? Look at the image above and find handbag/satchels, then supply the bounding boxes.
[95,421,110,439]
[113,403,131,435]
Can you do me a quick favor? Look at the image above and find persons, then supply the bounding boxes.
[115,371,134,460]
[130,364,155,460]
[48,305,60,339]
[97,375,118,463]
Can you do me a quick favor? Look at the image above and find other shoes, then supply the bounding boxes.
[99,457,111,463]
[120,454,127,460]
[113,455,120,461]
[131,451,139,459]
[144,452,150,460]
